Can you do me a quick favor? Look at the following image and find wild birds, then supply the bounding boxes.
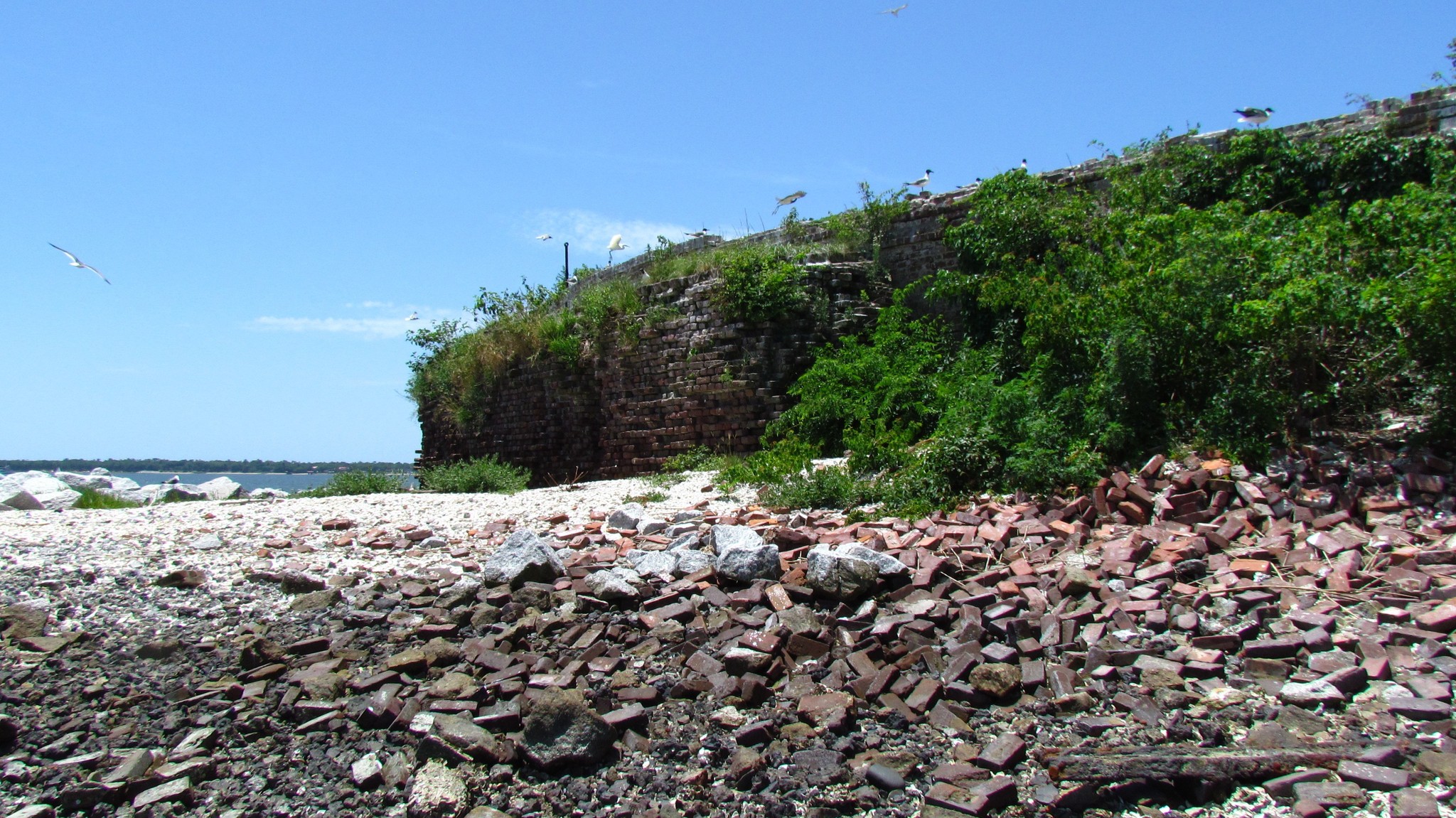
[684,229,709,238]
[903,169,934,191]
[536,234,552,241]
[879,4,909,17]
[1232,108,1276,129]
[772,190,807,215]
[1009,158,1028,170]
[956,178,982,189]
[607,234,630,259]
[405,311,420,322]
[639,268,651,281]
[48,242,111,285]
[565,275,578,286]
